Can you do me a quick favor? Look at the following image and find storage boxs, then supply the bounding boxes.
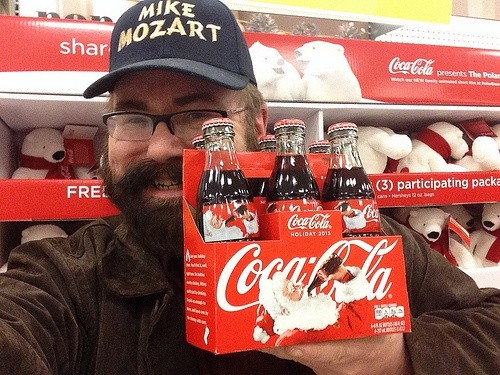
[182,148,410,355]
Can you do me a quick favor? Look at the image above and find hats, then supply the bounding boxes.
[83,0,257,99]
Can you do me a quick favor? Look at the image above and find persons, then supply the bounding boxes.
[0,0,500,375]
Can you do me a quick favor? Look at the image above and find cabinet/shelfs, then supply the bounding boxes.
[0,16,500,290]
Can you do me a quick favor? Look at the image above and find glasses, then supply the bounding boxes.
[102,107,250,142]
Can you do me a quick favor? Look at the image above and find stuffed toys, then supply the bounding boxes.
[11,127,76,178]
[0,224,69,275]
[395,121,468,173]
[441,205,479,242]
[393,207,419,228]
[408,208,474,268]
[463,203,500,267]
[355,125,413,174]
[453,124,500,171]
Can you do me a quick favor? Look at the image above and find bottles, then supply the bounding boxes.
[192,133,205,150]
[257,134,277,152]
[265,117,323,213]
[323,122,382,239]
[307,253,342,293]
[196,117,261,243]
[307,139,330,153]
